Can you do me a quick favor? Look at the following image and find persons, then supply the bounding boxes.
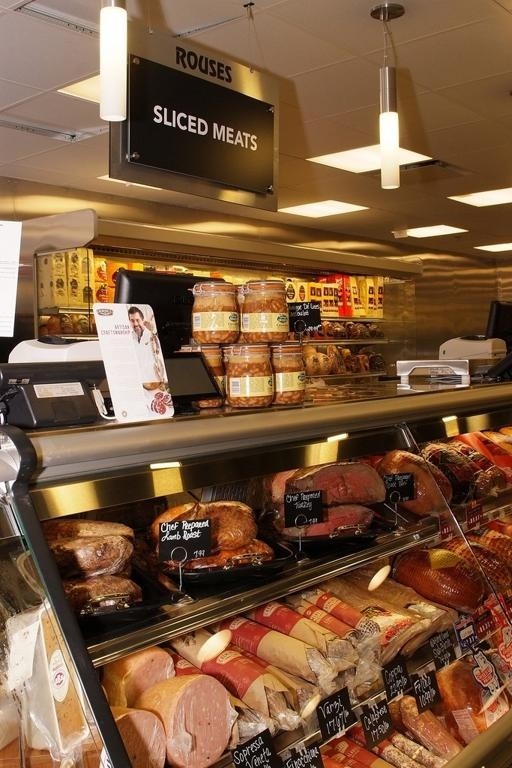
[126,307,161,407]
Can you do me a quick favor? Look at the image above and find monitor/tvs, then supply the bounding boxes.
[114,270,225,358]
[485,301,512,338]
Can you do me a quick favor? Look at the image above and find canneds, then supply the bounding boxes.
[181,279,305,406]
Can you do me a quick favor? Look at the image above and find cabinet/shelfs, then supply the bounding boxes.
[2,376,512,768]
[301,316,388,381]
[41,305,100,343]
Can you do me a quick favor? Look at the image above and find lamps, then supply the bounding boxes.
[370,2,405,190]
[98,0,131,124]
